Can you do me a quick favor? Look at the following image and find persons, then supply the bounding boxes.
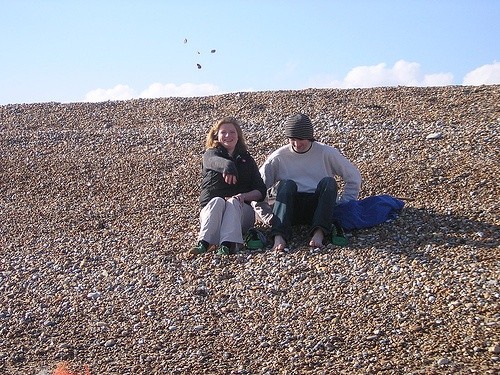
[258,112,362,253]
[189,116,267,256]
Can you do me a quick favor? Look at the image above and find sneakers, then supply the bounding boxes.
[190,239,209,254]
[215,241,235,255]
[330,224,349,247]
[243,227,267,250]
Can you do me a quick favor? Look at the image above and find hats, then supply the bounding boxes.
[285,113,314,140]
